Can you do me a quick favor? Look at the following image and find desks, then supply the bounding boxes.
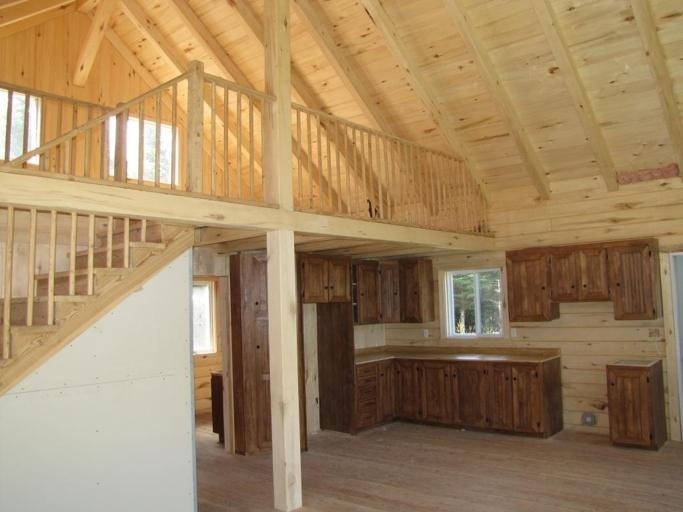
[210,372,224,443]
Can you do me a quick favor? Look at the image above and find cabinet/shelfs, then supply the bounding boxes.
[550,245,610,303]
[353,358,563,439]
[298,254,353,303]
[505,248,559,322]
[353,261,400,324]
[230,253,308,455]
[607,361,667,451]
[399,260,435,324]
[608,238,663,320]
